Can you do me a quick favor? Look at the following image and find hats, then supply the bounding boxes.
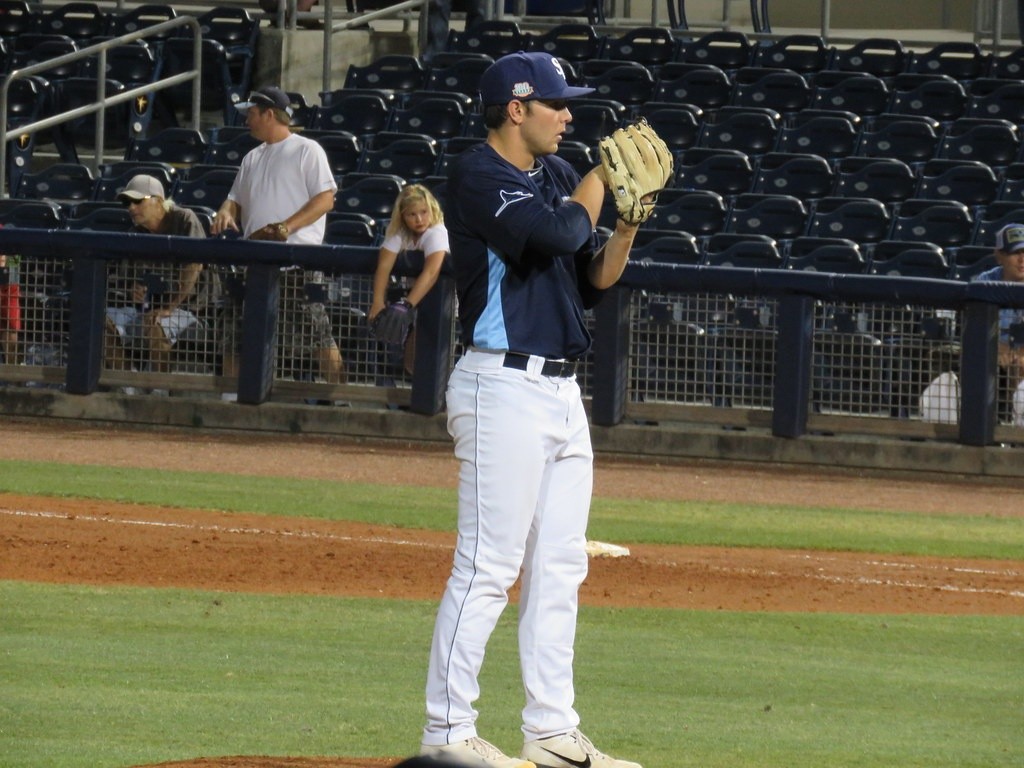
[116,175,164,200]
[995,224,1024,254]
[233,86,294,118]
[478,50,596,103]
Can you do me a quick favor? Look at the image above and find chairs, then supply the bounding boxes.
[0,0,1024,423]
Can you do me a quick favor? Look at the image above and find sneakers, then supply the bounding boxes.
[520,730,643,768]
[420,735,537,768]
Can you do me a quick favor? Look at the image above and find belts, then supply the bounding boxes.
[464,348,577,378]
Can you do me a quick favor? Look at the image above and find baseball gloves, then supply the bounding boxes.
[370,297,415,347]
[246,222,288,243]
[598,118,674,225]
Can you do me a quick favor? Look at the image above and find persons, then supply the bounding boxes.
[919,224,1024,427]
[209,87,351,406]
[367,184,459,413]
[420,52,674,768]
[103,174,221,397]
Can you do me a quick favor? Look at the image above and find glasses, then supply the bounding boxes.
[246,90,276,104]
[123,198,144,206]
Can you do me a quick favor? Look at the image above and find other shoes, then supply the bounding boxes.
[330,398,352,407]
[118,366,146,395]
[221,391,238,403]
[151,387,170,397]
